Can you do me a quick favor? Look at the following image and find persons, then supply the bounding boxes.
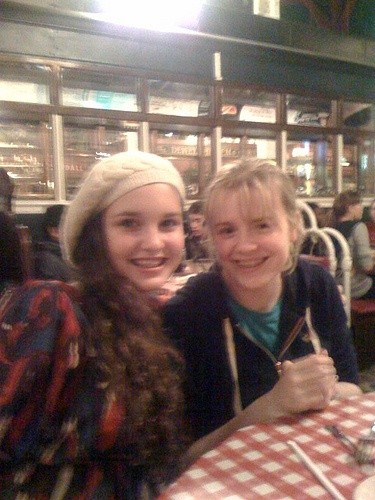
[184,201,208,259]
[0,152,339,500]
[159,156,362,439]
[35,204,74,280]
[303,190,375,300]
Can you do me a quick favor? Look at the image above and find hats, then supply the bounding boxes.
[62,151,185,269]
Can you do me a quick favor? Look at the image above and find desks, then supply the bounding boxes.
[68,258,216,305]
[155,389,375,500]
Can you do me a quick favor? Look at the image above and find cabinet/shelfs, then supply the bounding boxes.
[0,120,363,200]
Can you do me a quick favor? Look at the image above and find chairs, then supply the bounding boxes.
[0,209,33,286]
[297,199,375,330]
